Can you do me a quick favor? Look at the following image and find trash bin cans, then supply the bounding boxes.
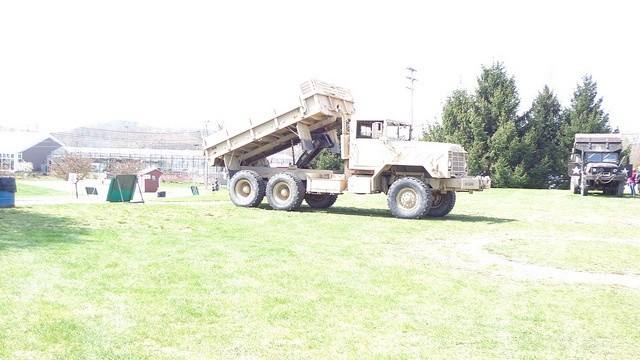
[1,176,16,207]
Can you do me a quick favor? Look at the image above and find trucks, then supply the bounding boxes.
[569,133,625,196]
[201,81,492,219]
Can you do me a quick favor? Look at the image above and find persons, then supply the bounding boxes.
[636,176,640,195]
[629,170,636,197]
